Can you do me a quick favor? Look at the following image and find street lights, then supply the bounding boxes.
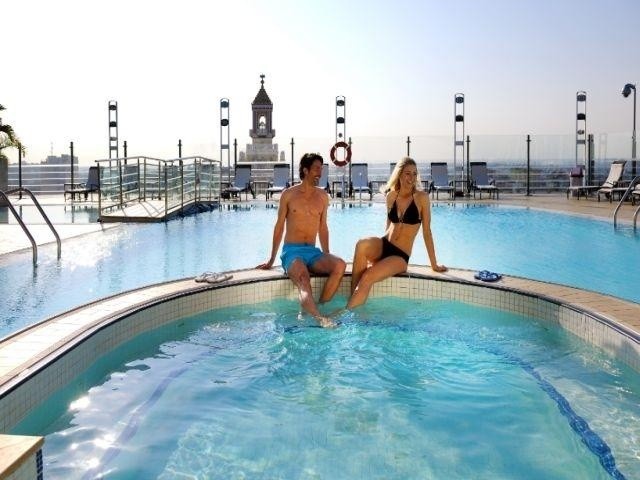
[621,83,637,180]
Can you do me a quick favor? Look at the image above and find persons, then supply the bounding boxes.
[254,152,347,327]
[331,157,446,320]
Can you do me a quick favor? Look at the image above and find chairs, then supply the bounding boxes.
[349,163,372,200]
[430,162,455,201]
[318,164,328,195]
[220,164,255,202]
[265,164,290,204]
[469,162,499,201]
[567,159,640,206]
[63,163,216,203]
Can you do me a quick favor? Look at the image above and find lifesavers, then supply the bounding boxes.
[330,142,351,164]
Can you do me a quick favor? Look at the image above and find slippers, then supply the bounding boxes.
[475,271,501,281]
[196,272,233,282]
